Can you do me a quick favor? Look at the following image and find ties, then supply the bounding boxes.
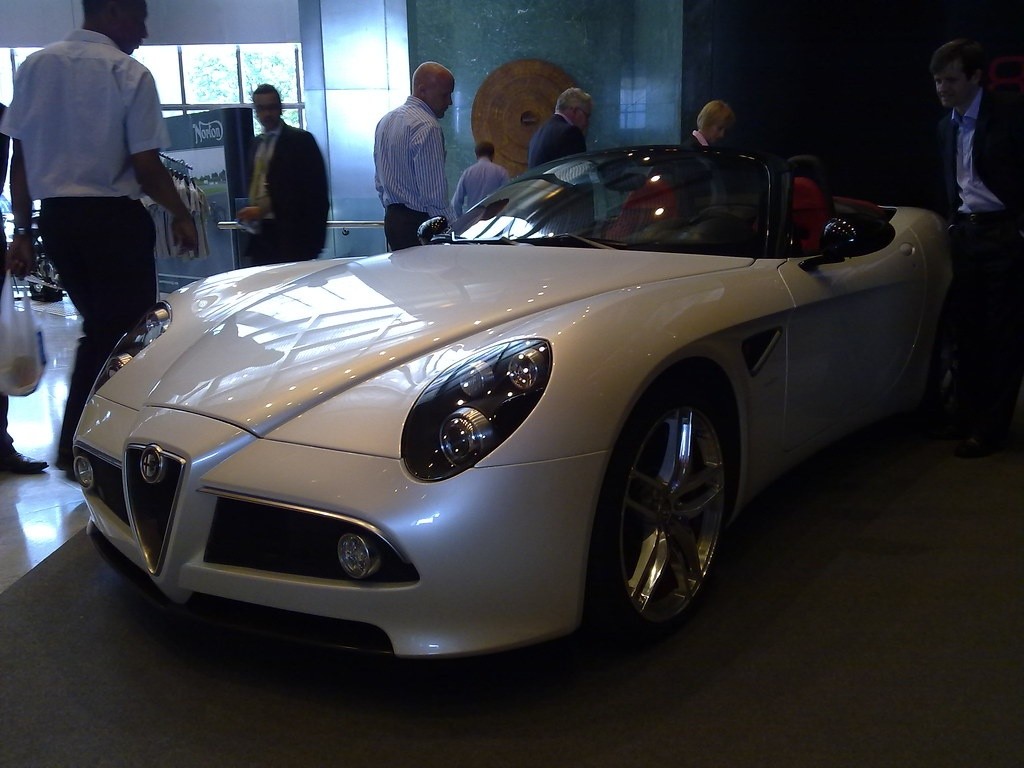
[249,133,271,205]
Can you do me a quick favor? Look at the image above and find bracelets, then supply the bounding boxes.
[14,226,30,235]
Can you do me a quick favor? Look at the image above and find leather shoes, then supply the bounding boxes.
[952,434,1008,458]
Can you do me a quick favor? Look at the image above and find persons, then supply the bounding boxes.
[527,87,596,238]
[0,104,51,472]
[0,0,200,474]
[926,38,1024,459]
[238,84,330,270]
[371,60,456,251]
[451,141,509,221]
[661,96,736,210]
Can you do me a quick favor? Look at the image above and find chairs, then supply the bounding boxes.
[750,156,836,256]
[602,170,696,243]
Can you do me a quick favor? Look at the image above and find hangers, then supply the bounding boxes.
[159,151,199,189]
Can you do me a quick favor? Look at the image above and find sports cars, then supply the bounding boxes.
[71,142,957,660]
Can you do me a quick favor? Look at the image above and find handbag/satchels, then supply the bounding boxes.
[0,269,47,397]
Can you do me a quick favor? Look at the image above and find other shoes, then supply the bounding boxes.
[0,445,49,474]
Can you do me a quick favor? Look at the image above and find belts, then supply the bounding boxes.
[957,210,1008,222]
